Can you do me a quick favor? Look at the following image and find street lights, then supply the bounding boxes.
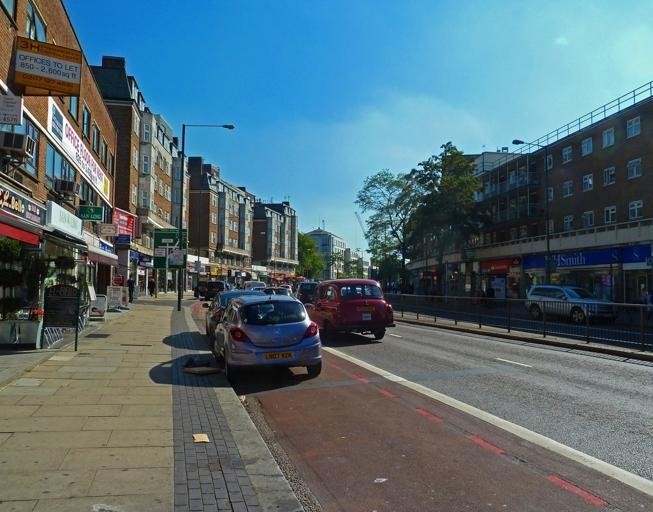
[512,139,551,285]
[178,124,235,311]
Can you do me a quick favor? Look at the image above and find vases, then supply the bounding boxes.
[30,314,42,320]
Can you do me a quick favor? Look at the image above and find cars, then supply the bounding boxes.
[194,279,395,384]
[524,284,618,324]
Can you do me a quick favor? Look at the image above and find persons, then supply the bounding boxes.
[91,307,104,316]
[147,279,156,296]
[126,274,135,303]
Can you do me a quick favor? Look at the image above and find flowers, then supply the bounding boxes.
[29,308,44,315]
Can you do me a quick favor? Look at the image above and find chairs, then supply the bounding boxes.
[60,306,83,332]
[78,305,90,332]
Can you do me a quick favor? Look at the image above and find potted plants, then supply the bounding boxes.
[0,295,27,319]
